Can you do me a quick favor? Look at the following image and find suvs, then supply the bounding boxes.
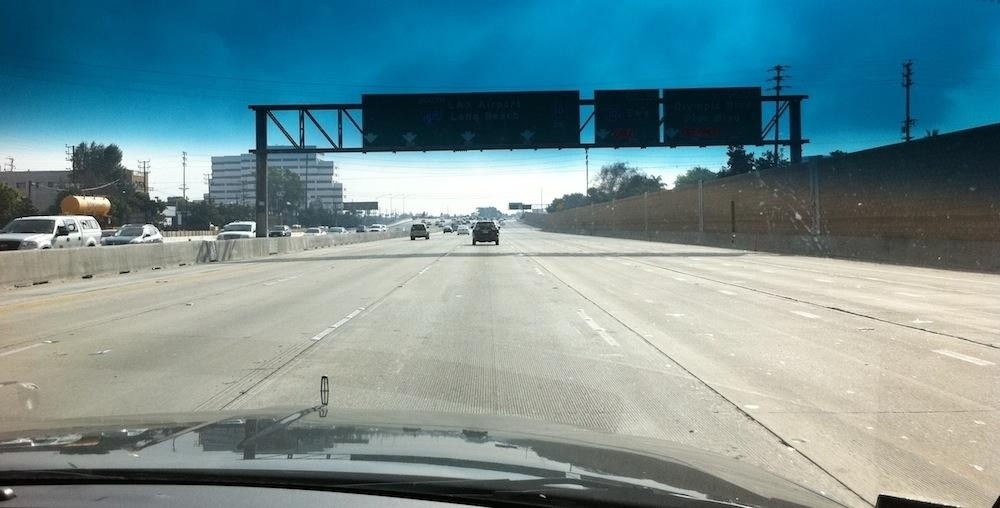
[269,224,291,237]
[471,219,500,246]
[410,224,431,241]
[216,221,256,241]
[104,223,163,245]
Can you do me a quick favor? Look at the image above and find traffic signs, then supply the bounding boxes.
[362,90,580,152]
[663,88,761,145]
[595,89,659,146]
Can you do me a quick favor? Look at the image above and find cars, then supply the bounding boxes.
[0,376,858,506]
[292,223,351,235]
[356,215,517,235]
[100,229,117,244]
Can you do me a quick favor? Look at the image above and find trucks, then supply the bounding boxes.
[0,211,103,253]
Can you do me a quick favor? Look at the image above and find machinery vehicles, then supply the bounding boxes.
[60,191,114,230]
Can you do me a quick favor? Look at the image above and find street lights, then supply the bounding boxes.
[347,202,409,224]
[585,146,591,204]
